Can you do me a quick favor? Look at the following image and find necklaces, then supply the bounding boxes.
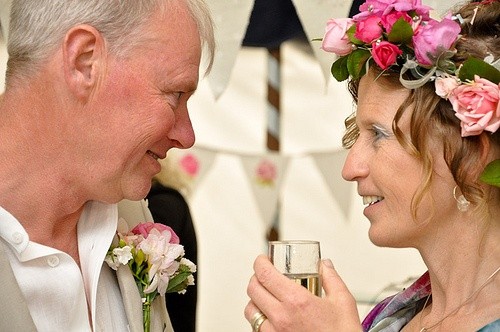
[419,267,500,332]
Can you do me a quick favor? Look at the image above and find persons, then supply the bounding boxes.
[1,1,216,332]
[244,0,500,332]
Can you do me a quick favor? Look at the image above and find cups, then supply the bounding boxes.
[267,239,323,300]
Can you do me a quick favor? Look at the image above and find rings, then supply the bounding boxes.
[250,311,267,332]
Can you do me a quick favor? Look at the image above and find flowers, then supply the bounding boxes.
[311,0,500,189]
[103,221,197,332]
